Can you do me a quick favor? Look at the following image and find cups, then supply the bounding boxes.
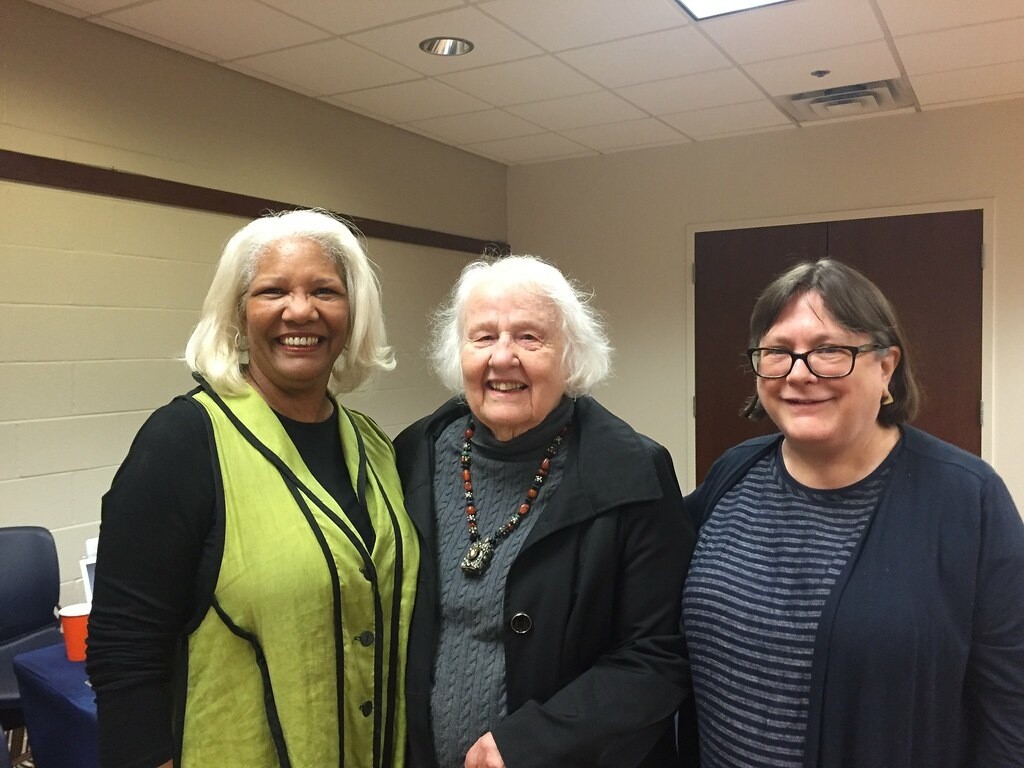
[58,603,93,662]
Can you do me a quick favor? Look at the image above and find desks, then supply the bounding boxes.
[13,642,100,768]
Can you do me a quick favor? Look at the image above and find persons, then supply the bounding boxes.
[85,208,421,768]
[684,256,1024,768]
[392,253,696,768]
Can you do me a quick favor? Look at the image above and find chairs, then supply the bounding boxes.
[0,525,63,761]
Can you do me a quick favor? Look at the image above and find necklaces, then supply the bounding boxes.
[460,415,565,577]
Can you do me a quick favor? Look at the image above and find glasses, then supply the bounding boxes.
[748,343,885,380]
